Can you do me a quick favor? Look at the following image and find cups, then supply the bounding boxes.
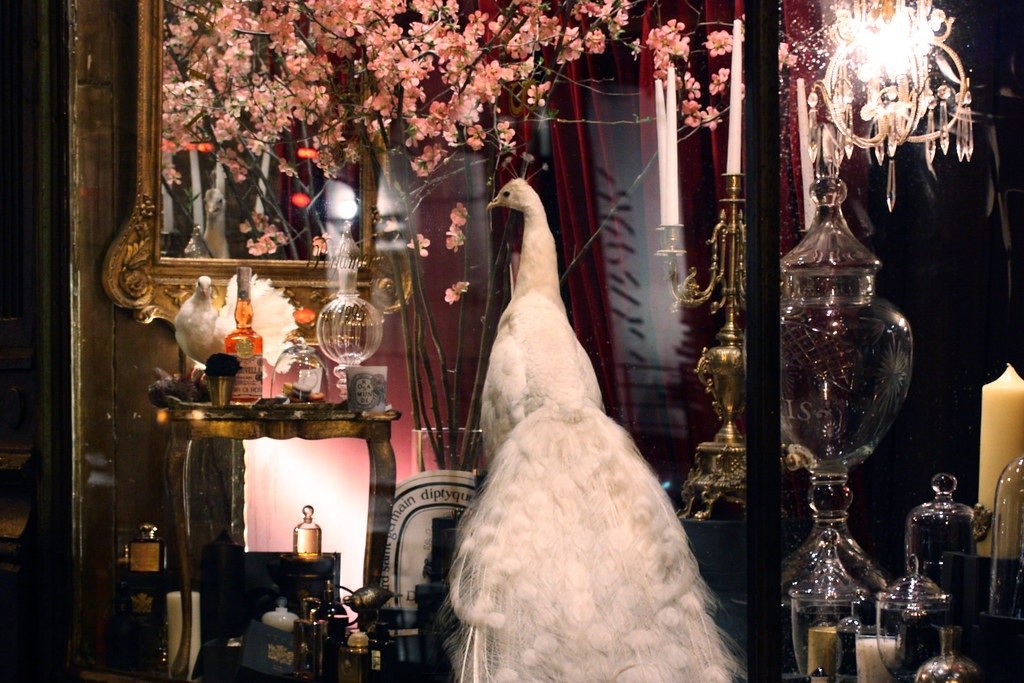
[206,374,236,407]
[905,473,978,630]
[855,634,896,683]
[270,345,330,403]
[788,530,869,676]
[875,554,952,683]
[989,457,1024,620]
[345,365,388,415]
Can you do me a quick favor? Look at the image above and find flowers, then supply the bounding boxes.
[164,0,756,469]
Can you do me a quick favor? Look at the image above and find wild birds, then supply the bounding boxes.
[448,173,734,678]
[173,273,299,378]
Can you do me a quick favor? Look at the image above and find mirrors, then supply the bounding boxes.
[96,0,377,331]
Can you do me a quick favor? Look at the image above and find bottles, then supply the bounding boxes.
[104,602,139,671]
[779,177,913,592]
[834,616,861,683]
[224,267,264,406]
[316,221,384,400]
[293,504,321,560]
[118,522,179,665]
[294,579,348,681]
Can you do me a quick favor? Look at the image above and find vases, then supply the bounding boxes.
[379,429,490,682]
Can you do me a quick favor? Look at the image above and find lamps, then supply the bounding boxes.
[797,0,974,212]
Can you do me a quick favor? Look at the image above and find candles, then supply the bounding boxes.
[858,634,898,683]
[215,153,226,193]
[727,19,742,172]
[974,363,1024,557]
[189,149,204,234]
[255,144,275,215]
[655,65,679,225]
[161,183,174,232]
[797,78,818,228]
